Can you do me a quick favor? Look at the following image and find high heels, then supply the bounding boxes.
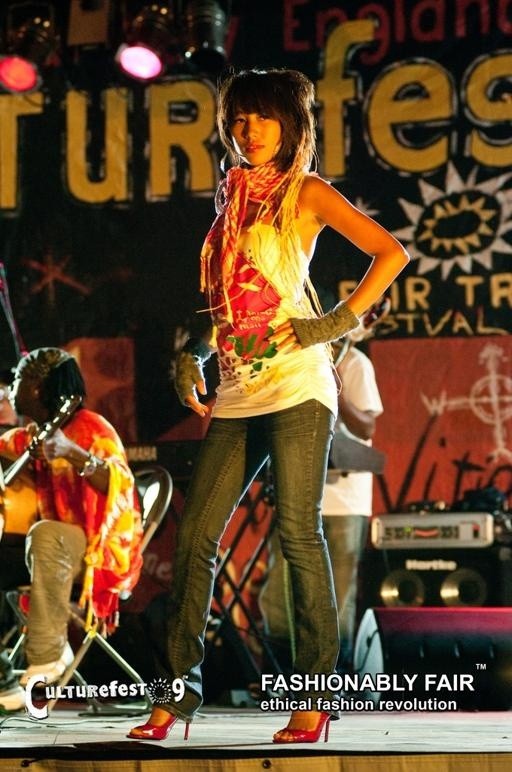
[272,709,332,744]
[126,701,191,741]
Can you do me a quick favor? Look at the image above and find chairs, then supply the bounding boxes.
[1,462,176,716]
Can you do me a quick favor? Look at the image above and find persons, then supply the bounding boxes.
[1,345,143,713]
[126,67,413,745]
[0,382,21,427]
[258,269,386,704]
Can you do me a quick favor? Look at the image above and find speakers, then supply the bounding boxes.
[353,607,512,712]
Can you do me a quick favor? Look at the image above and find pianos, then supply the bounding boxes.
[126,422,383,483]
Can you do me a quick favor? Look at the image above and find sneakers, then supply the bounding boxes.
[19,638,76,688]
[0,686,28,714]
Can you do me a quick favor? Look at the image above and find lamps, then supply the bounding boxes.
[1,0,231,96]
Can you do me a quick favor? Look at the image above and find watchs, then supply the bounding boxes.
[74,453,99,479]
